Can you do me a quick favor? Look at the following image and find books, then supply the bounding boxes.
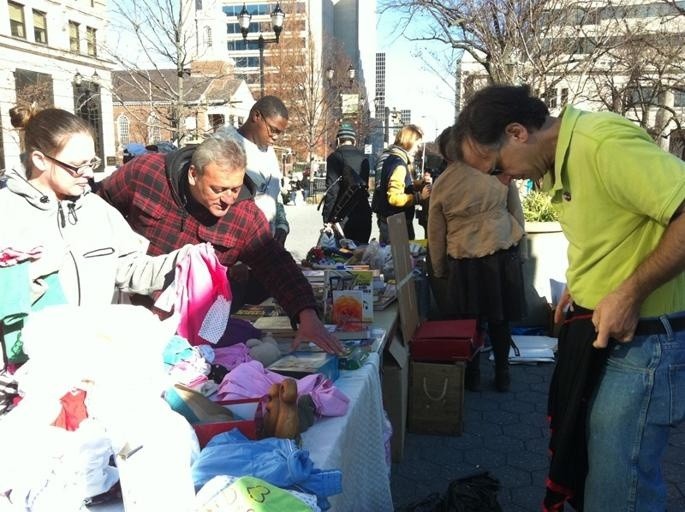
[301,265,397,310]
[253,315,297,334]
[232,302,284,322]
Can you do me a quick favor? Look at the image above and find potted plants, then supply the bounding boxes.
[519,188,572,309]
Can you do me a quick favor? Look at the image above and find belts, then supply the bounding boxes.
[635,316,684,335]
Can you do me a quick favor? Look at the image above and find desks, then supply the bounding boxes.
[83,352,381,512]
[330,302,402,353]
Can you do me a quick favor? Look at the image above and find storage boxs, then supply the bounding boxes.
[379,336,412,465]
[191,397,265,452]
[546,303,567,338]
[410,319,480,363]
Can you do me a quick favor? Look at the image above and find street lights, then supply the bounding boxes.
[325,62,356,126]
[238,3,287,98]
[73,66,102,127]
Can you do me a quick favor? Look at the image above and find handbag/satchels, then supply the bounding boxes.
[371,185,410,214]
[329,166,369,225]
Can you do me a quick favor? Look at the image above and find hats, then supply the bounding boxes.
[336,122,356,141]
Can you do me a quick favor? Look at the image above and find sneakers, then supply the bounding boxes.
[495,367,510,389]
[465,366,480,390]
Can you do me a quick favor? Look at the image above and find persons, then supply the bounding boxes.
[426,124,528,392]
[372,125,432,243]
[217,96,290,248]
[0,107,215,306]
[281,178,307,205]
[424,133,447,239]
[92,137,346,356]
[114,139,178,169]
[321,124,372,248]
[440,79,685,512]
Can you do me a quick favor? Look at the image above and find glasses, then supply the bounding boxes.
[40,149,103,176]
[255,107,286,140]
[488,140,504,176]
